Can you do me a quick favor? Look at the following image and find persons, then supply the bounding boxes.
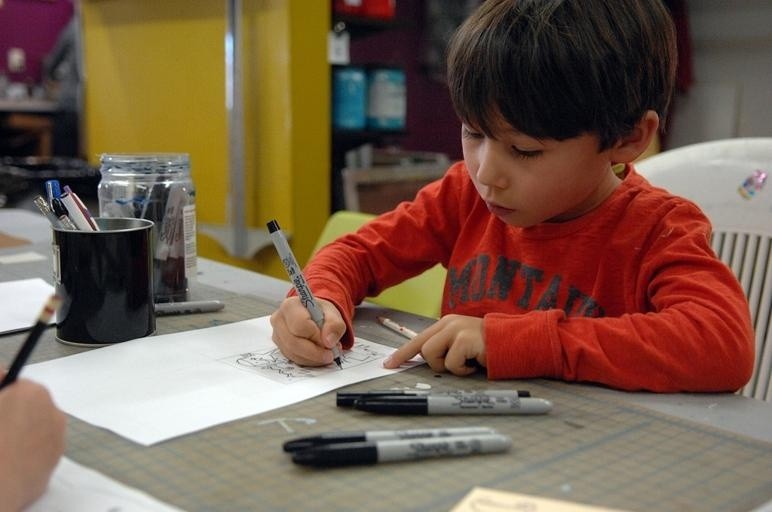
[0,371,67,511]
[268,0,757,396]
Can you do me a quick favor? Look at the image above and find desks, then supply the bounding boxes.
[0,208,772,512]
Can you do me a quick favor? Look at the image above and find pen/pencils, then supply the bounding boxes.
[266,220,344,370]
[0,294,63,388]
[154,300,225,315]
[377,317,418,339]
[33,180,102,233]
[283,390,552,465]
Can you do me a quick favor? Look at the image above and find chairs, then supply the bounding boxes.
[634,136,772,404]
[307,210,448,321]
